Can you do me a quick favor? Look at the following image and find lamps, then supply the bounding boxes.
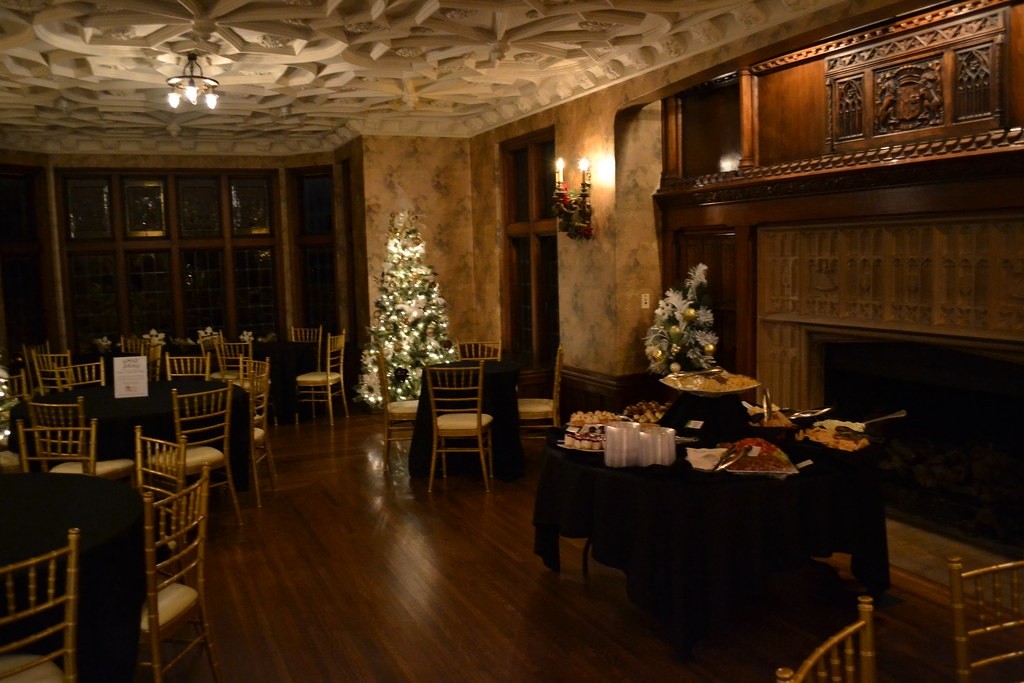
[166,53,220,109]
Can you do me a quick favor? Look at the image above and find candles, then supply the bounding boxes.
[580,156,588,183]
[557,157,565,182]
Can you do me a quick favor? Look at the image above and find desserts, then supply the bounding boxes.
[564,409,622,450]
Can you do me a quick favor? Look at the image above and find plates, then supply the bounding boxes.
[725,463,799,474]
[557,443,604,452]
[659,378,762,397]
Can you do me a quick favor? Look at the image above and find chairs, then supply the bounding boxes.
[948,553,1024,683]
[0,327,349,683]
[776,595,877,683]
[377,338,566,493]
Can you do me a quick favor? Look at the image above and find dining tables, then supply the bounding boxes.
[8,380,252,512]
[408,360,524,480]
[0,473,144,683]
[535,367,892,653]
[215,342,332,424]
